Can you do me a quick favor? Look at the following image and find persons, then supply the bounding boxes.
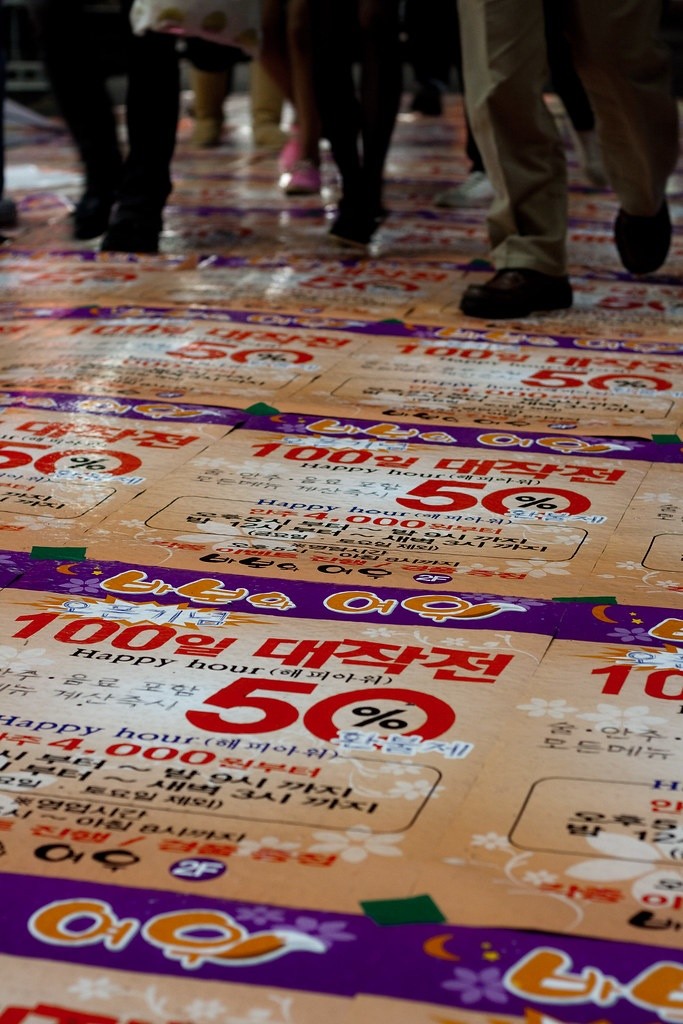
[0,0,683,321]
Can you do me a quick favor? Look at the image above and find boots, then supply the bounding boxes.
[248,65,288,150]
[312,62,401,247]
[188,75,225,150]
[45,33,180,253]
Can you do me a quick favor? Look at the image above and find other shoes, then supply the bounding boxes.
[613,188,673,275]
[459,267,574,319]
[666,174,682,196]
[372,224,409,242]
[460,171,493,201]
[287,121,323,197]
[0,199,16,227]
